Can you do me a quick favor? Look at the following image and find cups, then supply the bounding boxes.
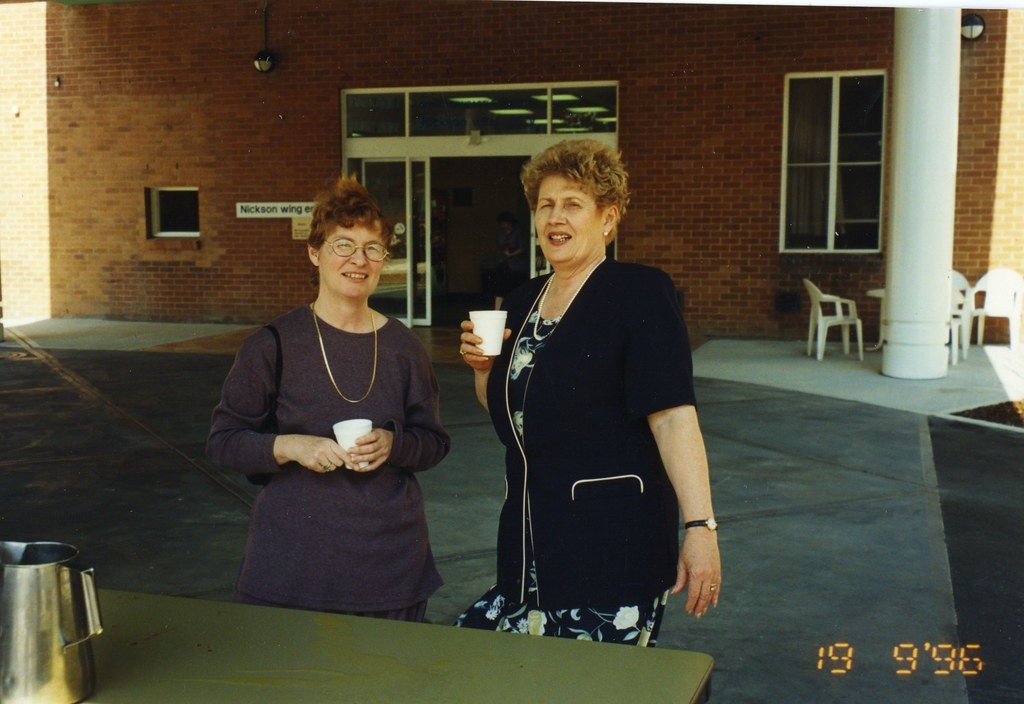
[468,310,507,356]
[333,419,373,469]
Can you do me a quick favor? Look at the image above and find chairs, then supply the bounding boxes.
[800,277,864,362]
[962,268,1024,360]
[950,269,975,366]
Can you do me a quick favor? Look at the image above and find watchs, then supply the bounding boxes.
[685,521,718,531]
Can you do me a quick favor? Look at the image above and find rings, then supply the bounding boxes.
[711,584,717,591]
[460,349,466,355]
[324,462,331,469]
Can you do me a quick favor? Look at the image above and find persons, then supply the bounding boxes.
[206,174,453,621]
[493,211,530,309]
[452,140,720,647]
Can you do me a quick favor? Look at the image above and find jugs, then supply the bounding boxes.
[0,540,104,704]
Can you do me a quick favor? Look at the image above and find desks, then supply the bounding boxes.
[87,585,717,704]
[864,289,888,351]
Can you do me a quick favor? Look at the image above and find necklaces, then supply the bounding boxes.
[312,300,377,403]
[534,256,606,341]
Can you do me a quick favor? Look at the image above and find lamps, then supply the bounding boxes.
[961,14,985,42]
[253,2,275,74]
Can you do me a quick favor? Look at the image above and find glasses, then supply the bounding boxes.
[324,237,390,262]
